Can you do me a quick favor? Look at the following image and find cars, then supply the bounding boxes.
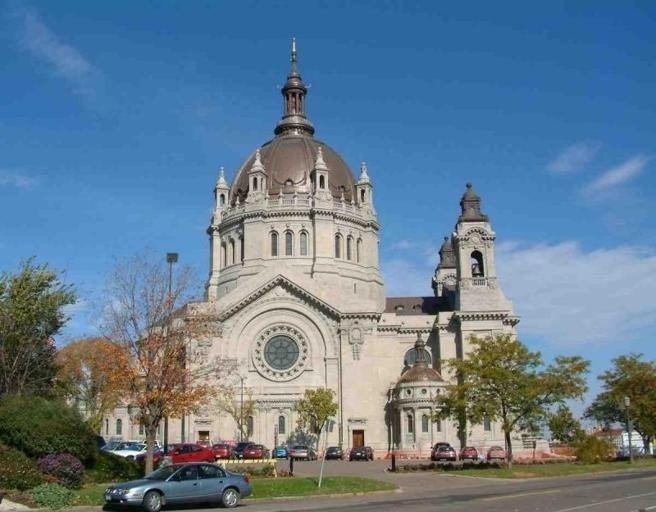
[272,448,288,459]
[101,440,269,464]
[430,443,456,461]
[106,463,252,512]
[459,447,478,461]
[487,446,506,460]
[290,446,315,461]
[349,447,373,460]
[327,447,343,460]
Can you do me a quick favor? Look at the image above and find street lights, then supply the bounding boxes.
[624,397,633,464]
